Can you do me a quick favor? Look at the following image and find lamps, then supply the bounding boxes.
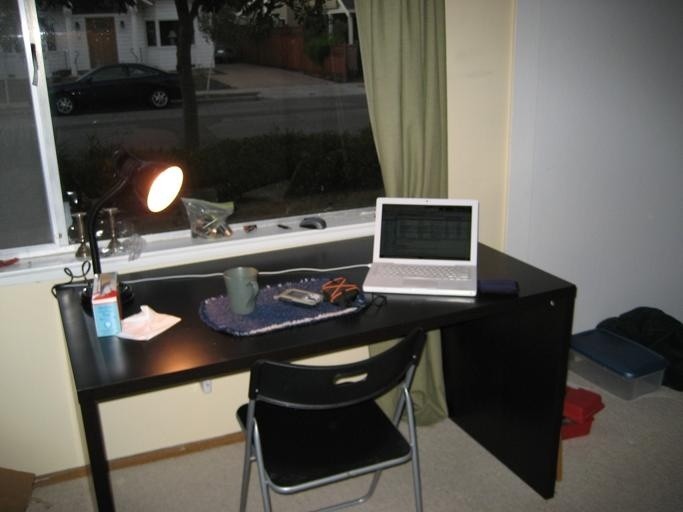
[80,146,182,320]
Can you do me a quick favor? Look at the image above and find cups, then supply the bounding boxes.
[222,266,260,316]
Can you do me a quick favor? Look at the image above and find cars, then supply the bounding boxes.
[42,61,181,116]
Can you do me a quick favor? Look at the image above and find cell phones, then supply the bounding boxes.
[280,288,324,307]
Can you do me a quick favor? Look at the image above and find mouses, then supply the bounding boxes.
[300,216,326,229]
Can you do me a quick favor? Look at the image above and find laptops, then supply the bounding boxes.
[361,197,479,299]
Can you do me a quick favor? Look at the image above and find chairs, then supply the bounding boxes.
[232,324,429,512]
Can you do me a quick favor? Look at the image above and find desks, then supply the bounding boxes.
[48,234,579,512]
